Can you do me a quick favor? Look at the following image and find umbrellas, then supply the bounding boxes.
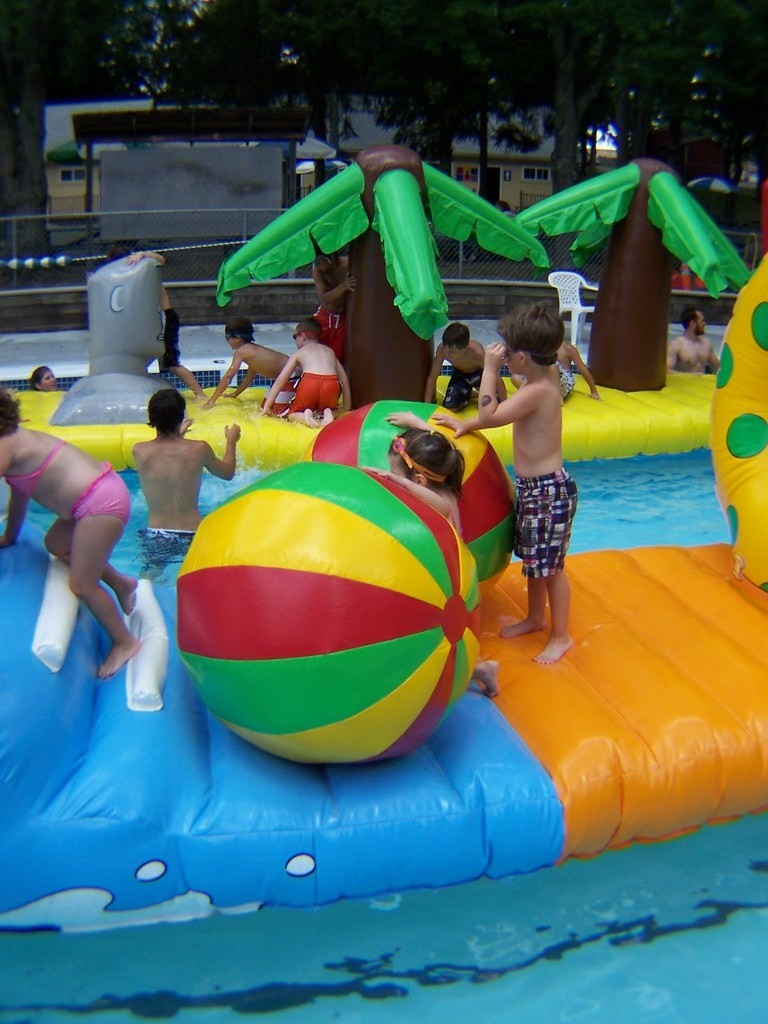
[687,175,742,216]
[238,135,354,214]
[46,139,153,165]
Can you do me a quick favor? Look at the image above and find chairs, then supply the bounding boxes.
[548,271,598,366]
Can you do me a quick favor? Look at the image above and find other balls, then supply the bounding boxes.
[177,459,480,766]
[301,400,515,593]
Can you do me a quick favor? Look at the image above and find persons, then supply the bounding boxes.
[665,306,720,376]
[359,411,501,699]
[312,238,358,365]
[0,385,142,678]
[29,366,58,391]
[132,388,241,581]
[506,340,601,407]
[255,316,352,428]
[430,299,578,664]
[424,321,507,413]
[196,317,303,418]
[107,246,210,402]
[494,200,516,219]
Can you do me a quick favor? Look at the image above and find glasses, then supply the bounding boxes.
[293,331,321,340]
[392,436,446,483]
